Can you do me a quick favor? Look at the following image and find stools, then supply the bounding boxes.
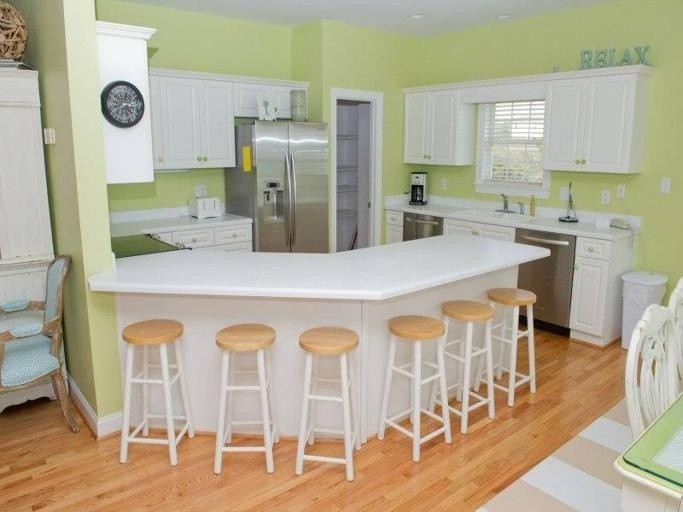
[213,323,280,476]
[473,287,538,407]
[116,316,195,469]
[373,312,453,462]
[427,298,498,434]
[293,325,362,481]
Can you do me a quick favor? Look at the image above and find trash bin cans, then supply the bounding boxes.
[620,270,669,352]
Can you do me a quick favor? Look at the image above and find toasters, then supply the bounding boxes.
[188,195,223,220]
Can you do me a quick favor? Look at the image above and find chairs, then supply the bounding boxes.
[623,301,682,442]
[0,252,81,438]
[666,276,682,385]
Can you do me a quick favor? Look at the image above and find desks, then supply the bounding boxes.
[84,232,552,443]
[614,388,683,512]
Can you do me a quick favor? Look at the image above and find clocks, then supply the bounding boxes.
[99,80,145,129]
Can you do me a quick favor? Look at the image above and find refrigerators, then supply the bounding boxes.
[235,119,331,253]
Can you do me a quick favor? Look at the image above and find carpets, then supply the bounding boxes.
[473,392,656,512]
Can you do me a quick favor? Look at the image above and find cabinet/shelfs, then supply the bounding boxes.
[335,131,358,222]
[91,20,158,187]
[171,219,254,252]
[400,82,475,168]
[567,230,641,349]
[0,66,71,419]
[145,228,175,246]
[384,208,403,246]
[161,68,236,171]
[147,66,164,175]
[541,63,657,175]
[442,217,516,243]
[231,74,311,121]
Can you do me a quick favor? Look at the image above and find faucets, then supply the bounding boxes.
[500,193,509,211]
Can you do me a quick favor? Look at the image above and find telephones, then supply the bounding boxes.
[609,218,630,229]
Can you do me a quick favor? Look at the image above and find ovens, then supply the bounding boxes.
[515,229,576,338]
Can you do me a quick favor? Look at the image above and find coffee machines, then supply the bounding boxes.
[407,172,429,205]
